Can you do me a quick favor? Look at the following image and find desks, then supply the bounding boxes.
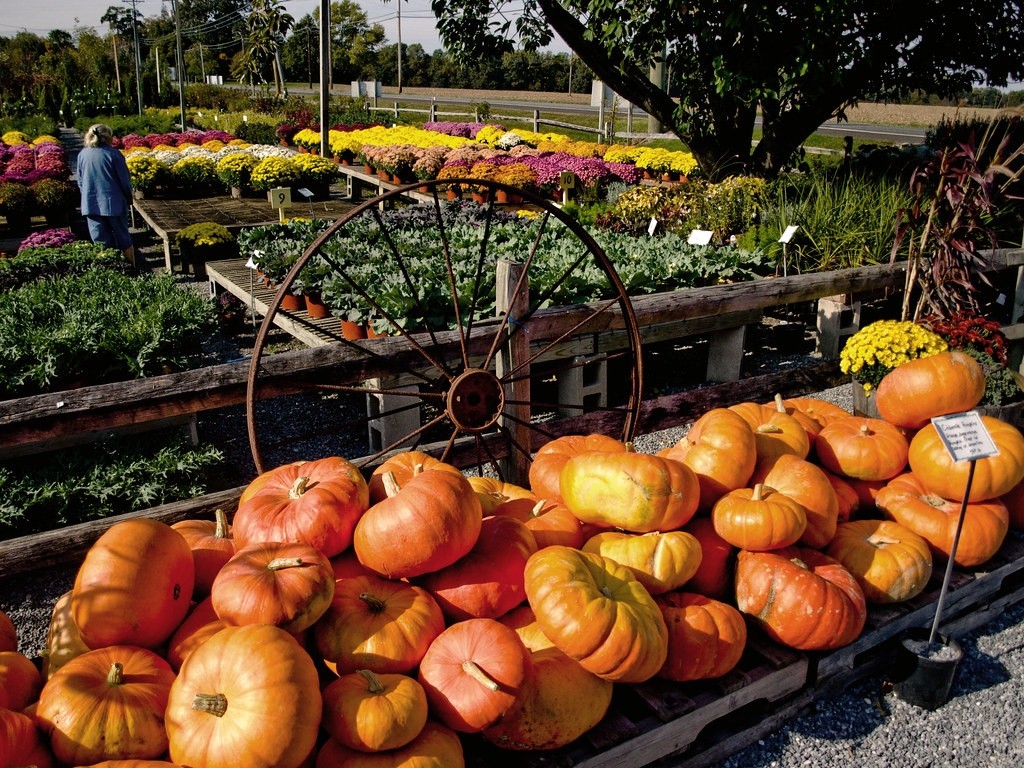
[204,258,751,465]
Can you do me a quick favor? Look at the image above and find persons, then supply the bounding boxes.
[77,124,135,266]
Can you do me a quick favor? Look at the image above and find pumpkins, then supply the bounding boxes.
[0,352,1024,768]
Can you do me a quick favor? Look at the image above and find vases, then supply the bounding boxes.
[365,164,377,175]
[302,147,310,153]
[382,173,393,181]
[280,138,293,146]
[511,194,524,203]
[661,172,673,181]
[193,184,209,194]
[298,146,303,153]
[311,149,318,155]
[193,256,220,281]
[447,189,463,201]
[643,170,654,179]
[342,158,353,166]
[134,188,153,200]
[231,186,250,199]
[552,191,562,202]
[377,168,382,177]
[333,156,343,163]
[678,174,690,182]
[497,191,511,203]
[417,179,436,193]
[305,182,329,199]
[470,192,487,204]
[393,175,406,184]
[849,376,1024,434]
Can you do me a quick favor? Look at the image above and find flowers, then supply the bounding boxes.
[839,307,1024,412]
[0,122,702,256]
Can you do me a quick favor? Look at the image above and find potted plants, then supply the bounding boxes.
[0,200,776,542]
